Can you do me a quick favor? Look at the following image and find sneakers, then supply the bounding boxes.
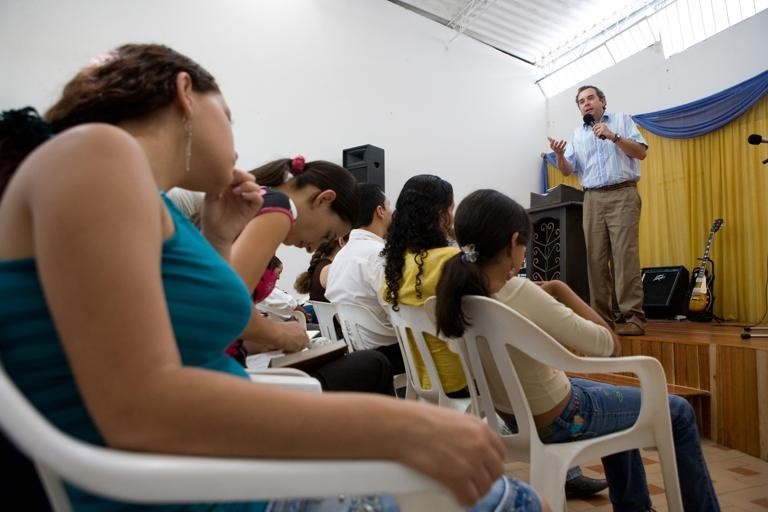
[617,322,644,336]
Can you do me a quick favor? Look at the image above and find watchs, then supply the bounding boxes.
[611,132,622,143]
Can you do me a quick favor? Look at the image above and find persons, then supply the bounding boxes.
[168,155,397,400]
[547,84,647,335]
[378,176,609,501]
[434,190,721,512]
[0,43,553,512]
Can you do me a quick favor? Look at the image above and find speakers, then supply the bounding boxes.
[342,144,385,190]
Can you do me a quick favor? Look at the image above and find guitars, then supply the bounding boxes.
[689,219,725,311]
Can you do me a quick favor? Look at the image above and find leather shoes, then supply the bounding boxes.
[566,475,609,500]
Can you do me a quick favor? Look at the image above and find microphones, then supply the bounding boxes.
[583,113,604,135]
[746,134,768,146]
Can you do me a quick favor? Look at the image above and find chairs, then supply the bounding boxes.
[304,298,342,342]
[329,300,407,397]
[0,359,466,511]
[378,300,484,413]
[424,294,685,512]
